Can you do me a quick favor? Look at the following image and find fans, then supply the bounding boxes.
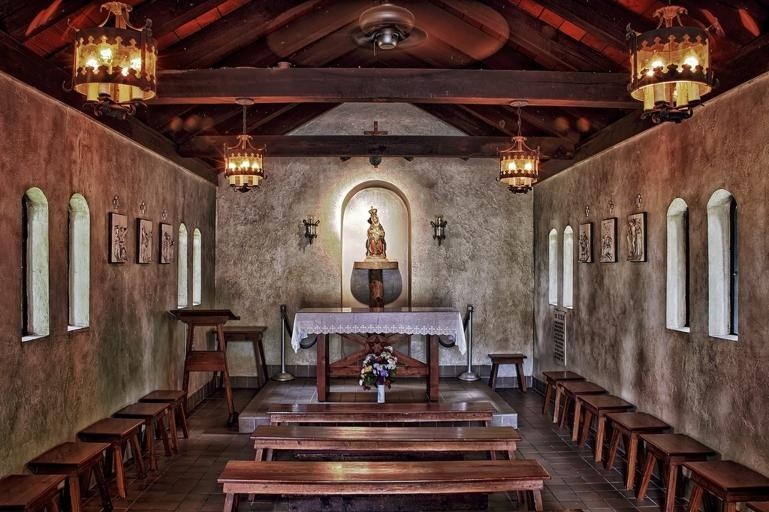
[267,1,512,70]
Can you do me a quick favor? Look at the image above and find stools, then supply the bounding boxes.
[0,388,191,510]
[542,367,769,511]
[208,324,271,389]
[486,350,529,396]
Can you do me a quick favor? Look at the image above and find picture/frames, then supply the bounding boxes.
[107,211,128,265]
[135,217,154,263]
[598,217,619,263]
[157,221,173,265]
[577,223,594,264]
[626,211,647,262]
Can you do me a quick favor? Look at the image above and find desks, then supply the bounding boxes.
[291,306,468,405]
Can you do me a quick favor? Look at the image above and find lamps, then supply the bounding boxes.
[62,0,159,122]
[496,100,542,195]
[625,1,720,124]
[302,218,320,246]
[430,215,447,246]
[222,96,271,194]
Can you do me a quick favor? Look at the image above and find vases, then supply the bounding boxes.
[377,384,386,404]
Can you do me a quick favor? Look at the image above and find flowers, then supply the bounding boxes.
[359,345,399,390]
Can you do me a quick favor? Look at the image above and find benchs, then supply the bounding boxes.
[264,401,498,459]
[216,457,549,512]
[246,427,524,503]
